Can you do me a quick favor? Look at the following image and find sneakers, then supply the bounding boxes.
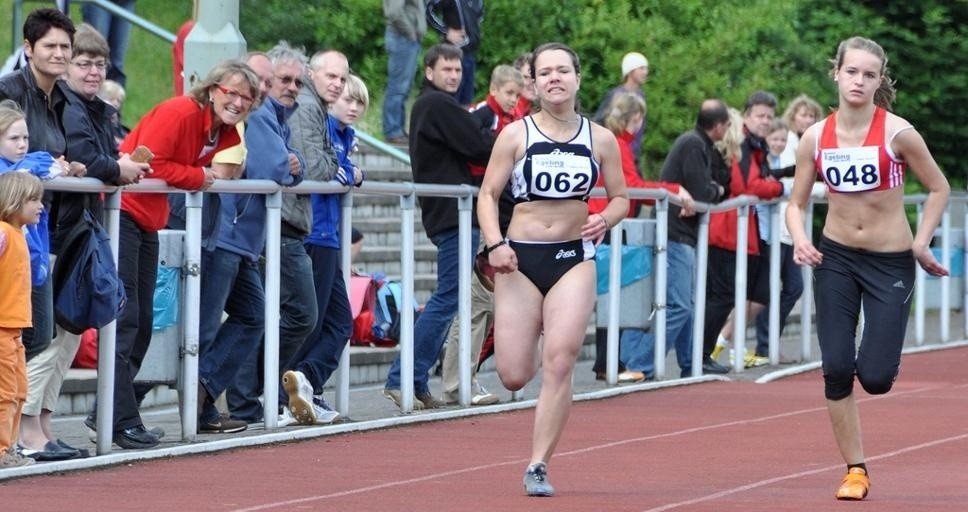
[710,342,724,360]
[522,462,554,497]
[79,413,164,449]
[728,348,772,369]
[201,416,248,434]
[417,391,441,409]
[595,368,646,385]
[278,402,341,427]
[472,385,500,406]
[197,381,207,435]
[835,466,871,500]
[779,354,798,365]
[282,370,317,424]
[0,451,35,469]
[383,386,425,410]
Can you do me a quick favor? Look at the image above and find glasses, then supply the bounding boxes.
[276,74,306,88]
[71,59,107,68]
[216,83,253,105]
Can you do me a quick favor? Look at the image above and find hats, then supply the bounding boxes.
[621,51,648,77]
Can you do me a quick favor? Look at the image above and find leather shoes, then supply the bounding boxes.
[16,441,82,460]
[679,357,728,378]
[56,438,89,458]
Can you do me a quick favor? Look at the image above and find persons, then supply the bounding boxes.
[786,35,952,501]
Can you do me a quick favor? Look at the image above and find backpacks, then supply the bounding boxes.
[52,192,127,335]
[369,277,422,343]
[349,275,376,345]
